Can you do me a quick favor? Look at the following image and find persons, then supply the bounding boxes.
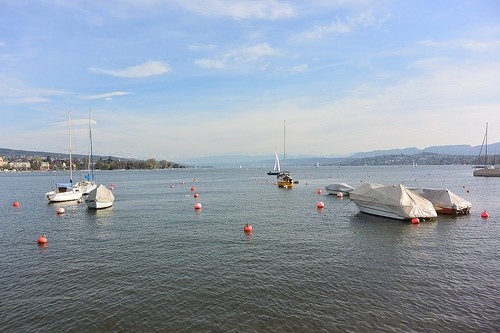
[269,171,271,173]
[283,175,288,181]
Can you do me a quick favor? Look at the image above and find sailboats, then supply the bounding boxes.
[44,108,83,202]
[71,108,97,196]
[266,119,294,189]
[472,120,500,177]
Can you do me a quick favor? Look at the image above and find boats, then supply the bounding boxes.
[325,182,356,196]
[84,183,115,210]
[348,181,437,223]
[406,186,472,216]
